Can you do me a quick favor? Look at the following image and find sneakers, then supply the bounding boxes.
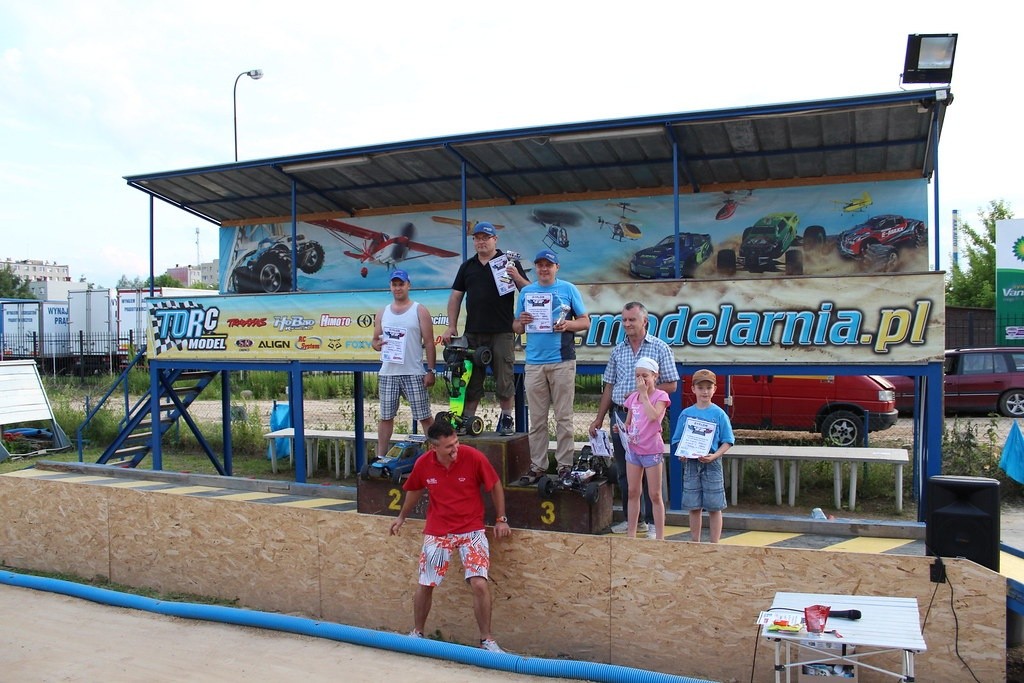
[408,630,422,637]
[480,639,506,654]
[611,521,649,534]
[499,414,514,436]
[645,523,658,539]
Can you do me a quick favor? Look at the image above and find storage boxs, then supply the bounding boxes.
[797,647,859,683]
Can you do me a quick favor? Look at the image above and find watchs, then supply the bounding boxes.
[427,368,436,374]
[495,515,508,523]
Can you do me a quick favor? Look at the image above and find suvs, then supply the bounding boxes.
[714,211,827,277]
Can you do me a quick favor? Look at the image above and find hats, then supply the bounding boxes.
[692,369,717,384]
[635,357,659,373]
[473,222,497,236]
[534,250,559,264]
[390,269,410,283]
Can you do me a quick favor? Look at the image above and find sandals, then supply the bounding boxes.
[519,469,544,486]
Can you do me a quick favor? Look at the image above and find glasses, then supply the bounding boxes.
[473,235,494,240]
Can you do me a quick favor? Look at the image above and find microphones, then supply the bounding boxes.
[828,610,862,619]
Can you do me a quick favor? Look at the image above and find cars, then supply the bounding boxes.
[359,440,426,486]
[835,214,925,263]
[880,345,1024,420]
[669,375,899,447]
[628,232,715,279]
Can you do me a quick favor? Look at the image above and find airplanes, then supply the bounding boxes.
[307,219,461,279]
[431,215,505,238]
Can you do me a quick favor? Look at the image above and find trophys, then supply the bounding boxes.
[555,304,571,329]
[500,250,521,284]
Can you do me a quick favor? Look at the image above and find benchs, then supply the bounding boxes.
[547,437,909,512]
[264,427,424,480]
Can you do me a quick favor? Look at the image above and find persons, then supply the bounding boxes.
[372,269,436,465]
[440,222,533,435]
[672,369,735,543]
[589,301,679,539]
[513,250,590,486]
[388,419,512,653]
[612,357,671,539]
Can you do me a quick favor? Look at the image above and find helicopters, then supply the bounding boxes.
[710,190,753,221]
[531,208,572,255]
[595,202,643,244]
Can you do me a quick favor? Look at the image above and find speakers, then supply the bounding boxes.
[926,475,1000,574]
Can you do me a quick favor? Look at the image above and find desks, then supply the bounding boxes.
[762,591,926,683]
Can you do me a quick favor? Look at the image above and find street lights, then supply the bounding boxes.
[232,68,264,163]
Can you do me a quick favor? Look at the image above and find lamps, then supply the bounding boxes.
[899,32,958,91]
[281,154,372,174]
[549,124,666,145]
